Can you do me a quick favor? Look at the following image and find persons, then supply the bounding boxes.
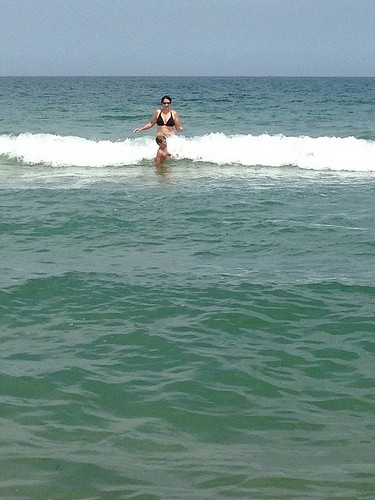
[153,136,172,165]
[133,95,184,137]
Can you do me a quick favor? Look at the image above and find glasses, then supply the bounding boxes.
[163,102,170,104]
